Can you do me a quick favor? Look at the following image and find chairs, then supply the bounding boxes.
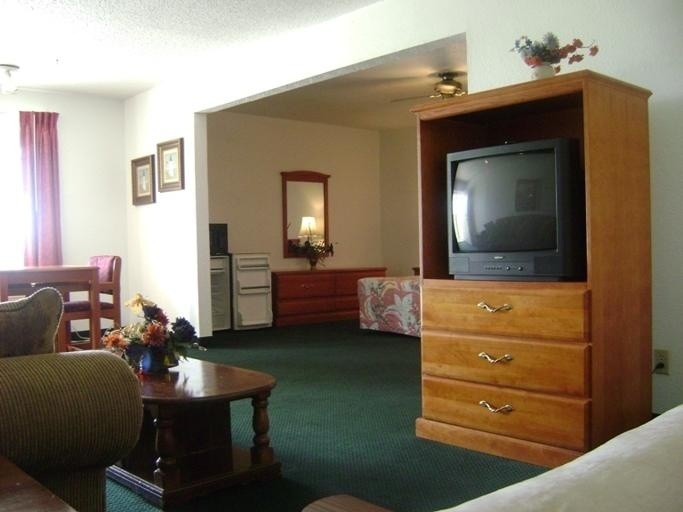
[59,254,120,353]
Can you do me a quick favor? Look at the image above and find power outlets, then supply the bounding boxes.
[651,348,667,374]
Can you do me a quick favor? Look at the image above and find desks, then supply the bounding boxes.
[1,268,99,353]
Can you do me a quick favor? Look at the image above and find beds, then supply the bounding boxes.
[356,268,421,342]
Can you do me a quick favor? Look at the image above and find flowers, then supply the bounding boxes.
[99,295,206,368]
[509,31,599,72]
[297,239,335,269]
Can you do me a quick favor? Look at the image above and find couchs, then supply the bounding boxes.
[1,290,146,511]
[299,404,682,511]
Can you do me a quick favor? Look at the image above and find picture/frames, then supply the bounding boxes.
[130,154,156,206]
[156,137,187,192]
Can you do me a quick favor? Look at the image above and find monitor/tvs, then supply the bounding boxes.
[208,223,228,257]
[447,137,586,281]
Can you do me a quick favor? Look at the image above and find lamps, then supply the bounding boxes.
[428,72,466,100]
[298,216,317,246]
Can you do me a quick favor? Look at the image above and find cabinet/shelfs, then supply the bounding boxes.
[271,267,387,326]
[408,69,653,467]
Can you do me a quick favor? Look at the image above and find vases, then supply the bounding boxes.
[530,61,553,77]
[137,346,166,374]
[309,255,317,269]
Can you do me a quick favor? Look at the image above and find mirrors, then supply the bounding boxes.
[279,170,332,258]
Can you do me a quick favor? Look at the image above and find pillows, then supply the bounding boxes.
[1,287,64,360]
